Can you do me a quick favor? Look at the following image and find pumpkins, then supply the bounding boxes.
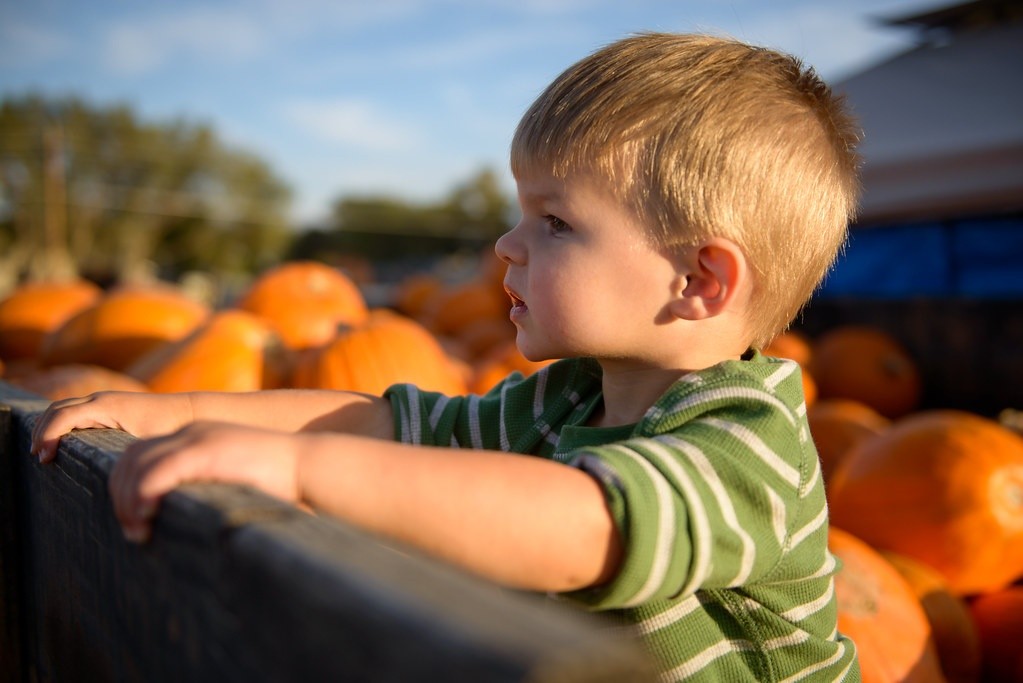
[0,261,563,412]
[753,325,1023,683]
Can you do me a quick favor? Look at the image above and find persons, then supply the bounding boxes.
[29,32,866,682]
